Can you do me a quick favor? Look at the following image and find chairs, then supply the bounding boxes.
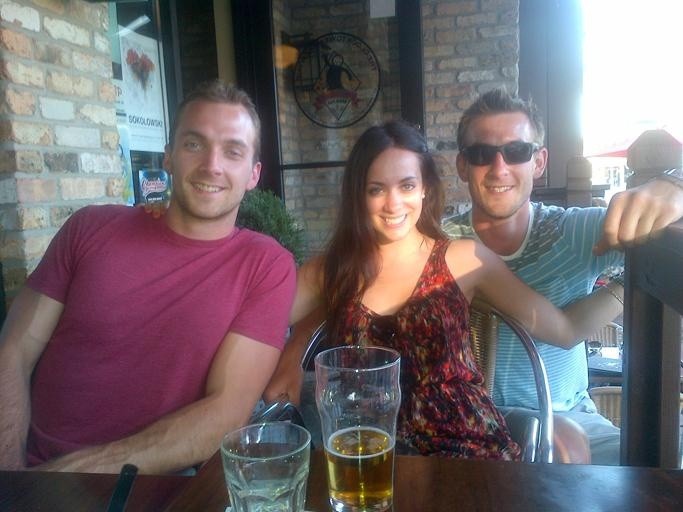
[288,296,554,464]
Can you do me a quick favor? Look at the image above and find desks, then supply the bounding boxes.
[0,446,682,510]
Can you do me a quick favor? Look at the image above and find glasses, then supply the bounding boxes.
[465,139,541,166]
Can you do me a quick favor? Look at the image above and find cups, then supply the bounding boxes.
[220,420,313,512]
[313,345,401,512]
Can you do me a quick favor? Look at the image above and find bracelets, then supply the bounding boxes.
[602,281,625,305]
[610,272,625,290]
[644,168,683,188]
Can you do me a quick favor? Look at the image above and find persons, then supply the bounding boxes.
[0,78,296,480]
[133,119,625,464]
[261,88,683,469]
